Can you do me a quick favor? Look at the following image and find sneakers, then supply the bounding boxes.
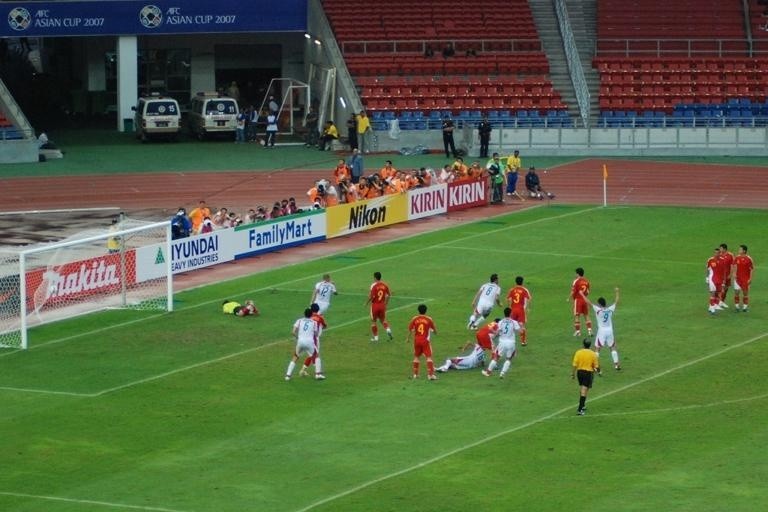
[284,376,290,380]
[315,374,325,379]
[615,365,621,369]
[574,330,581,336]
[582,406,586,409]
[371,335,378,342]
[386,328,393,340]
[300,370,308,376]
[587,331,593,336]
[577,410,584,415]
[413,320,528,380]
[708,301,748,314]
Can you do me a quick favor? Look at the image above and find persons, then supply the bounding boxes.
[443,42,455,60]
[477,117,493,159]
[234,95,372,154]
[170,198,296,240]
[424,45,434,58]
[465,46,477,59]
[704,243,755,315]
[441,115,461,158]
[362,271,395,343]
[284,272,340,382]
[404,266,635,416]
[307,149,544,206]
[220,297,260,318]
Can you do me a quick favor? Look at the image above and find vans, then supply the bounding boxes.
[185,91,240,143]
[130,95,182,143]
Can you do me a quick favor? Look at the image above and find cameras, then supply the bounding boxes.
[314,204,319,208]
[274,207,278,210]
[291,203,295,206]
[259,215,265,218]
[207,223,210,227]
[339,179,347,187]
[417,174,424,182]
[259,207,262,211]
[235,219,243,223]
[282,204,286,208]
